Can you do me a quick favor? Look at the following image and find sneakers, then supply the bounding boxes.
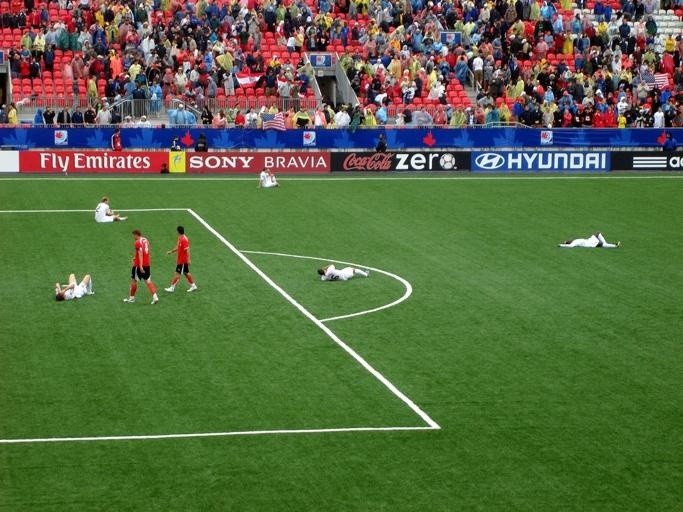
[186,284,198,293]
[151,297,159,305]
[123,297,135,302]
[164,287,174,292]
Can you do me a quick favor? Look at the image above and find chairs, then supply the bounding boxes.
[576,8,635,37]
[220,33,317,109]
[0,0,105,108]
[653,8,683,53]
[388,73,472,116]
[329,37,362,57]
[516,52,581,72]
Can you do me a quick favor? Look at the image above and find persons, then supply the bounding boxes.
[663,132,677,152]
[95,196,128,223]
[557,231,624,248]
[123,230,159,305]
[55,273,95,301]
[317,264,370,281]
[111,128,123,151]
[195,133,208,152]
[170,135,181,151]
[256,167,280,188]
[163,225,198,293]
[376,132,387,153]
